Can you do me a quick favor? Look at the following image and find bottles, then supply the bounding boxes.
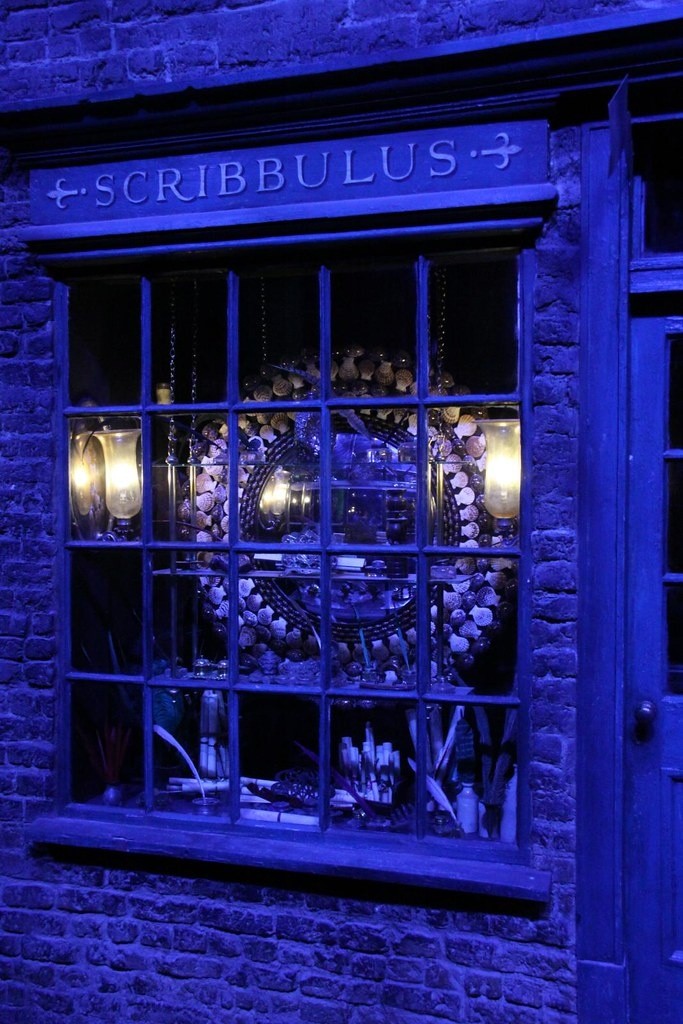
[500,763,518,844]
[456,772,480,833]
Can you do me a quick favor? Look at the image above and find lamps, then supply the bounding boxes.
[88,417,142,519]
[471,405,521,519]
[68,418,100,516]
